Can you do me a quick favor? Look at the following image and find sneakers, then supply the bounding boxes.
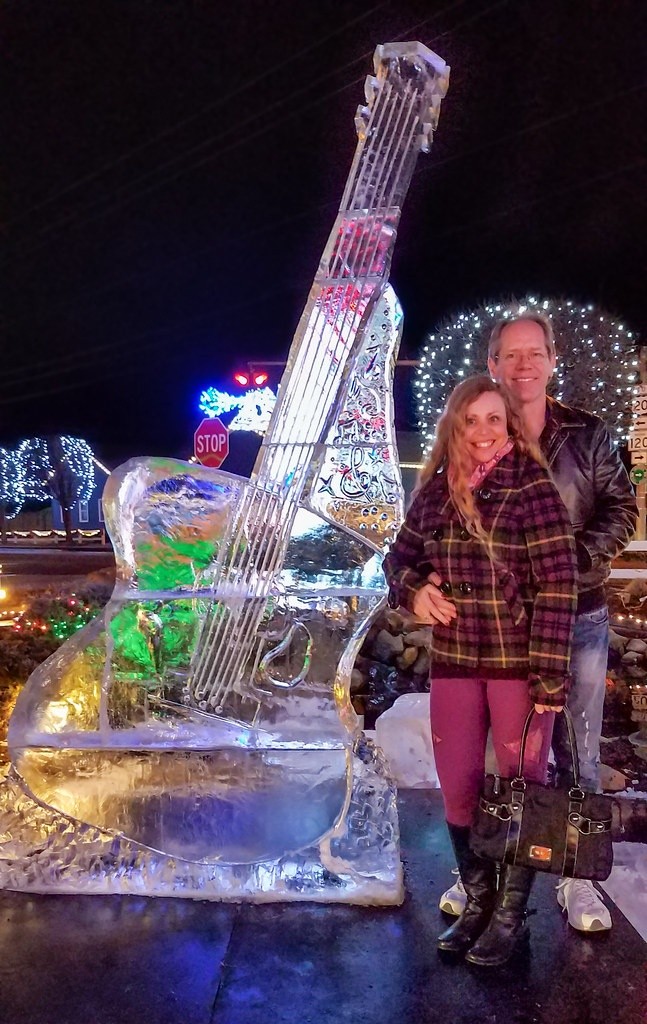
[439,867,499,916]
[557,876,612,932]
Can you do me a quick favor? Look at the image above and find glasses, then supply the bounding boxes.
[494,351,548,364]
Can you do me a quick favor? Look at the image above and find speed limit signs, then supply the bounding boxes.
[631,397,647,414]
[627,432,647,451]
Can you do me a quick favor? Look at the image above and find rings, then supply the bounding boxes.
[423,617,428,621]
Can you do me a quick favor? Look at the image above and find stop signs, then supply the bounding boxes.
[193,419,229,468]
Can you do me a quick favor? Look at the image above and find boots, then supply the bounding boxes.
[435,819,497,952]
[465,867,536,967]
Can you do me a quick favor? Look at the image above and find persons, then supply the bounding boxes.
[382,376,579,966]
[428,310,641,932]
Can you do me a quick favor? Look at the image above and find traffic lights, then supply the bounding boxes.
[235,374,268,387]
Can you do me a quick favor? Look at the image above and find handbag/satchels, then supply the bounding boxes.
[474,705,625,882]
